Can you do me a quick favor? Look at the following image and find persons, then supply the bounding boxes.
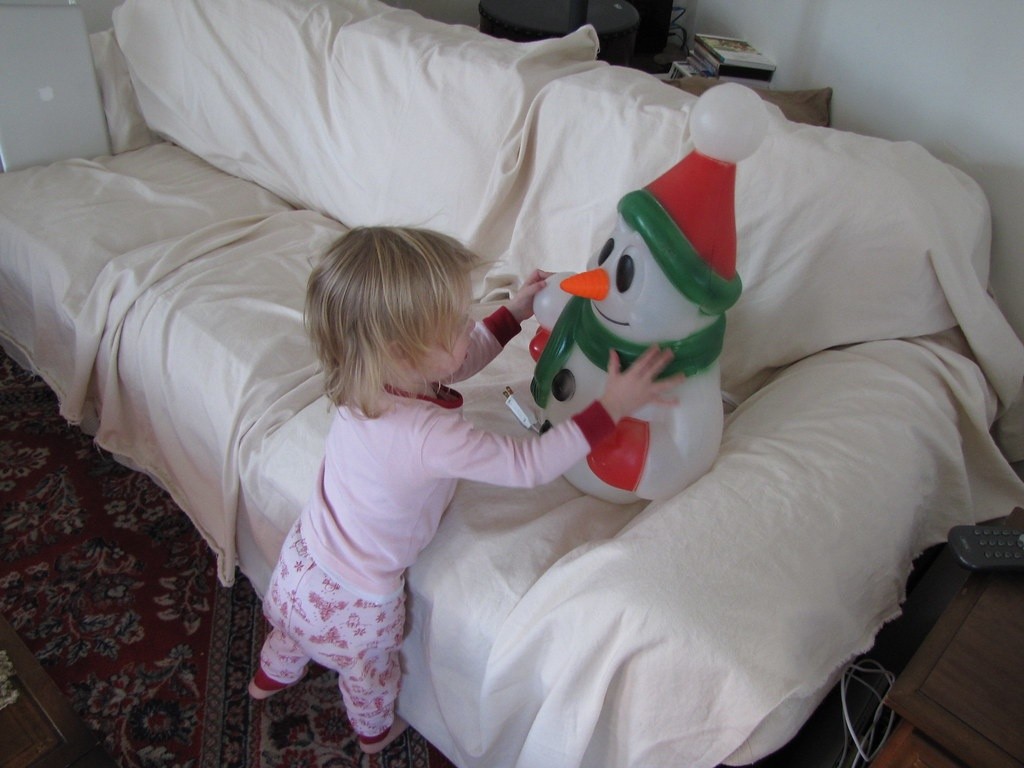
[247,226,684,756]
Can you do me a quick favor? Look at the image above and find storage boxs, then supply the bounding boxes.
[666,29,777,90]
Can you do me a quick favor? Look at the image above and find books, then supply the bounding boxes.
[665,32,776,93]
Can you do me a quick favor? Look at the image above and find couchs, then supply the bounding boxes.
[0,0,1023,767]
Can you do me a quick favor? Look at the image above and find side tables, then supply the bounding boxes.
[870,568,1023,768]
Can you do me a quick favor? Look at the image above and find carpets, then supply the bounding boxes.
[0,342,461,767]
